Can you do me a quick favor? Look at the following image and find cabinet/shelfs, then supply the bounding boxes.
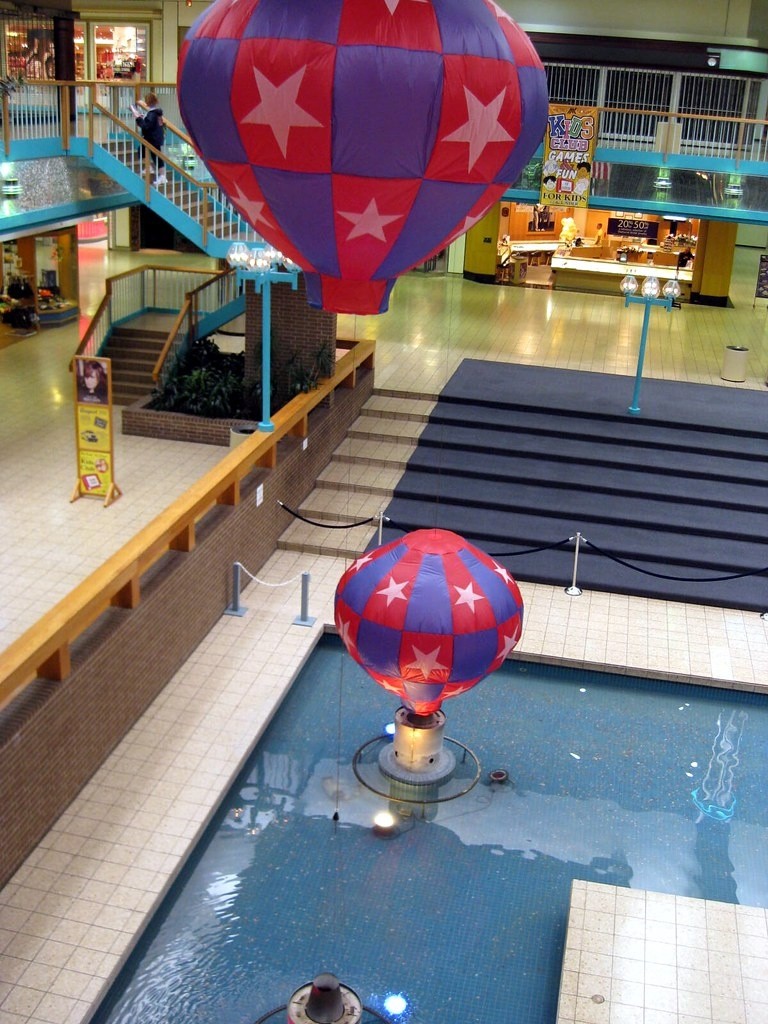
[7,25,148,83]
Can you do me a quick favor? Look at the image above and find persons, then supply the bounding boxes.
[594,223,603,246]
[540,206,551,230]
[575,238,584,247]
[125,53,131,62]
[78,361,108,405]
[130,56,142,82]
[127,93,169,184]
[111,55,122,72]
[532,204,541,231]
[678,246,694,267]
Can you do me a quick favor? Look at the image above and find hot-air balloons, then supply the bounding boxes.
[178,0,549,317]
[328,530,523,724]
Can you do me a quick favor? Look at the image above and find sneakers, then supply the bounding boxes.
[142,165,154,175]
[153,175,168,185]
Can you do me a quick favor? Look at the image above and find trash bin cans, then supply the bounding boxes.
[229,425,259,452]
[720,345,749,382]
